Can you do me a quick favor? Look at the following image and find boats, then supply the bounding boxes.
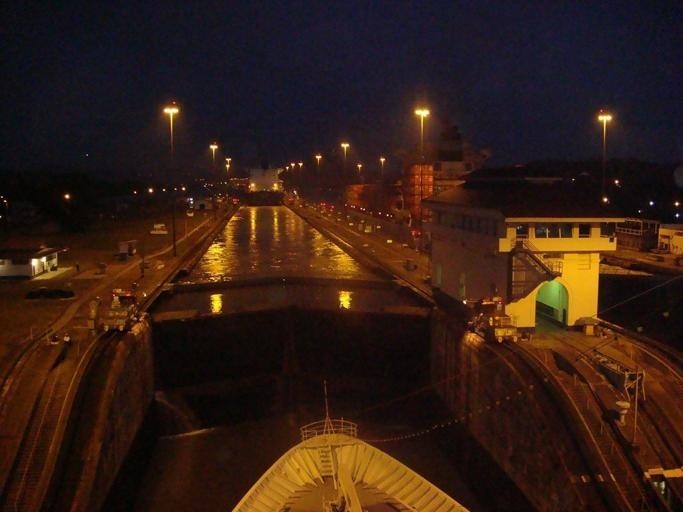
[232,434,470,512]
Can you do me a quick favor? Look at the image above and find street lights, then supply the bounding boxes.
[209,142,219,219]
[286,143,386,175]
[226,158,232,201]
[163,101,181,257]
[413,107,431,201]
[598,112,614,155]
